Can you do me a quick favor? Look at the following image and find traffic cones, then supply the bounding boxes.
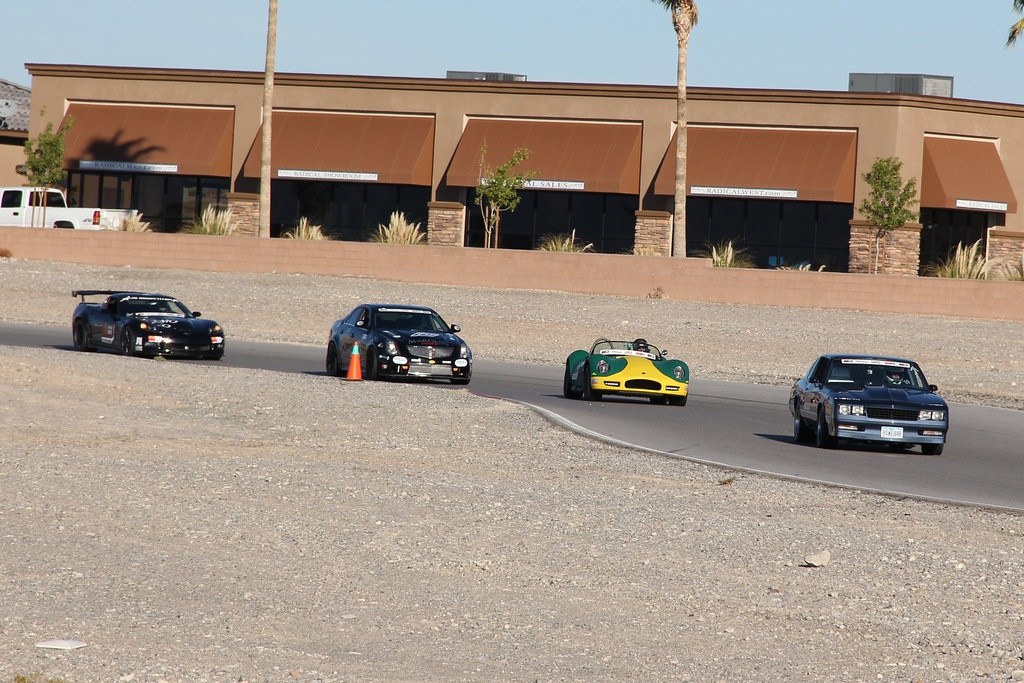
[340,340,365,382]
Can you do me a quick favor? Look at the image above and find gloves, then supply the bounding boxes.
[904,380,910,385]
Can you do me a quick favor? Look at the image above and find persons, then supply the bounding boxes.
[633,339,650,352]
[407,314,431,330]
[147,301,167,312]
[883,367,910,385]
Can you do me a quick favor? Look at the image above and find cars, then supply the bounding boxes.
[788,353,951,455]
[325,303,473,386]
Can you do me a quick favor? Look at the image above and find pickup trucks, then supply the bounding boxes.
[0,185,138,231]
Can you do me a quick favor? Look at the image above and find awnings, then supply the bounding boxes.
[243,112,435,186]
[53,103,234,177]
[446,119,642,195]
[654,127,857,204]
[920,137,1017,214]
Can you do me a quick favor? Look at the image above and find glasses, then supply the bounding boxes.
[638,346,646,349]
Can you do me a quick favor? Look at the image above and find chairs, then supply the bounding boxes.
[831,365,850,378]
[589,337,614,355]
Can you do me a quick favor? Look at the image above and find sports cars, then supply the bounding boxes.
[564,337,690,407]
[70,289,226,361]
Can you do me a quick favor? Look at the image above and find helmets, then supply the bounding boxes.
[884,366,904,385]
[632,339,648,350]
